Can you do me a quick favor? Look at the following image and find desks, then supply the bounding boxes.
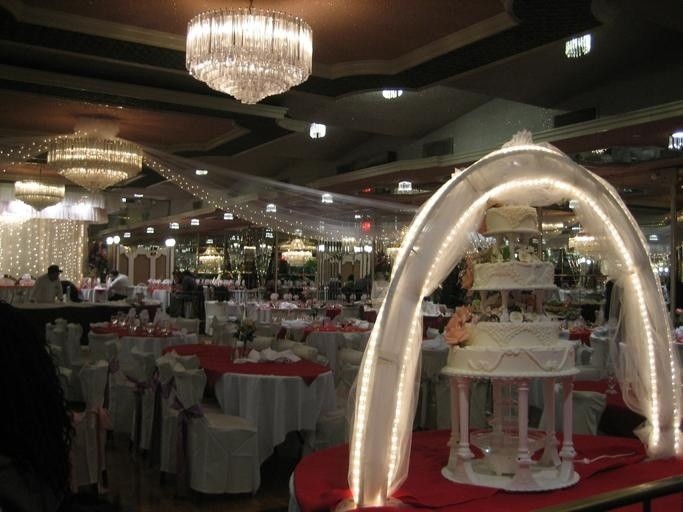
[291,428,683,512]
[363,302,683,436]
[369,302,683,427]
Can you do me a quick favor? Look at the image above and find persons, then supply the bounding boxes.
[28,264,64,303]
[169,271,205,321]
[0,300,78,509]
[103,269,128,301]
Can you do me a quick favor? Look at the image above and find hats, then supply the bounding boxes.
[46,265,62,273]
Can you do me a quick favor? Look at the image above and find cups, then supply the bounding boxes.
[108,311,172,340]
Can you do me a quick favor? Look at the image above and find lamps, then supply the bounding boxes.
[185,0,313,104]
[14,114,144,210]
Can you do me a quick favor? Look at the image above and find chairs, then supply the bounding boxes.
[46,279,375,496]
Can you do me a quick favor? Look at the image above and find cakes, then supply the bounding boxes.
[440,203,580,378]
